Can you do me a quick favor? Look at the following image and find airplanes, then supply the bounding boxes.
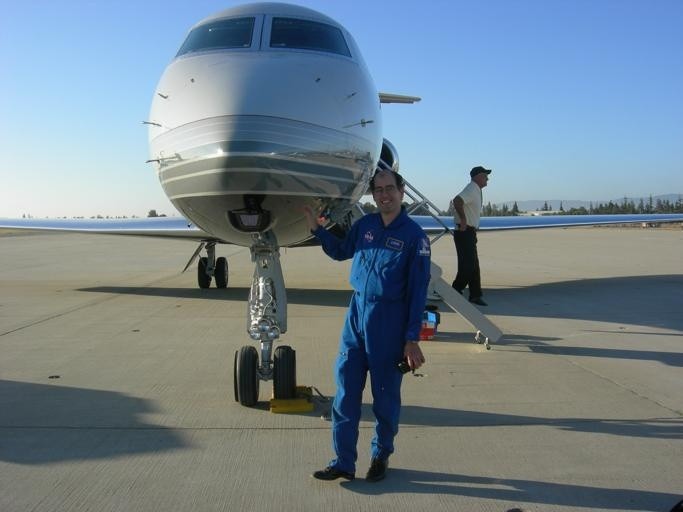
[0,0,683,406]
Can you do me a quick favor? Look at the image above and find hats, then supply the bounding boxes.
[470,167,491,178]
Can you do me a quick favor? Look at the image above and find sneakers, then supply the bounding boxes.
[366,457,388,482]
[469,297,489,306]
[311,466,355,480]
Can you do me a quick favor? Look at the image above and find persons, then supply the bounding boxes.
[450,165,493,307]
[300,168,432,483]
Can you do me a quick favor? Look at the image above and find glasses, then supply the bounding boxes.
[373,186,396,196]
